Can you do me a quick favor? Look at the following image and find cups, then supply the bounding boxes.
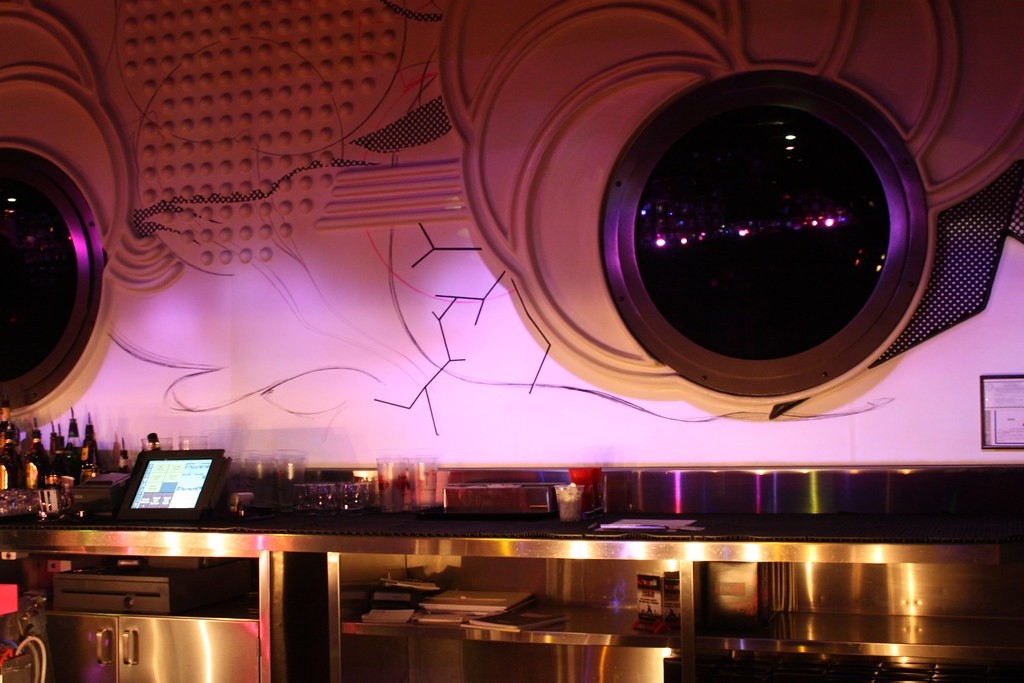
[377,457,406,513]
[407,457,440,511]
[178,436,208,450]
[555,484,585,522]
[244,458,276,509]
[274,455,307,511]
[141,438,174,450]
[297,481,369,518]
[569,466,603,512]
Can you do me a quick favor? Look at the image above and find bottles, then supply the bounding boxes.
[0,393,97,511]
[119,450,130,474]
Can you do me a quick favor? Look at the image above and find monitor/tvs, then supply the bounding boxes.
[118,448,225,522]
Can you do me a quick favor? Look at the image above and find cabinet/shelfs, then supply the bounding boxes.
[0,500,1024,683]
